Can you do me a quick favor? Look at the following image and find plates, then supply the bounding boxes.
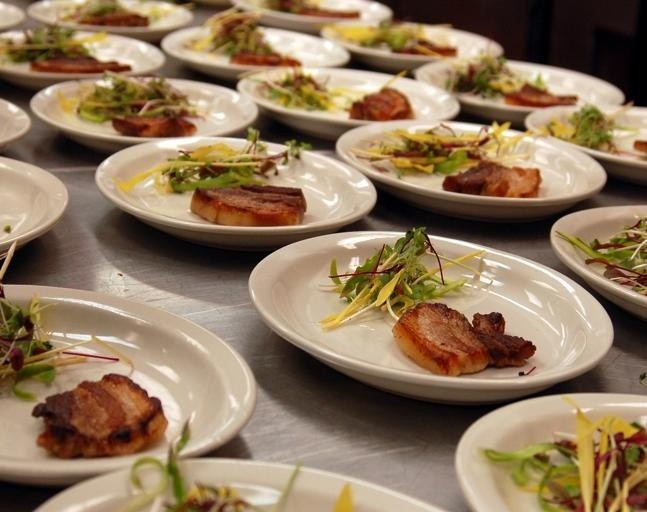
[524,104,647,178]
[31,458,445,512]
[95,138,378,247]
[28,78,259,148]
[337,121,607,216]
[0,31,164,84]
[248,231,615,401]
[0,157,69,252]
[321,21,504,72]
[0,285,257,480]
[229,0,387,37]
[552,206,647,315]
[415,59,625,119]
[0,99,32,144]
[0,2,24,27]
[238,68,460,136]
[454,393,647,511]
[161,25,348,78]
[27,0,194,38]
